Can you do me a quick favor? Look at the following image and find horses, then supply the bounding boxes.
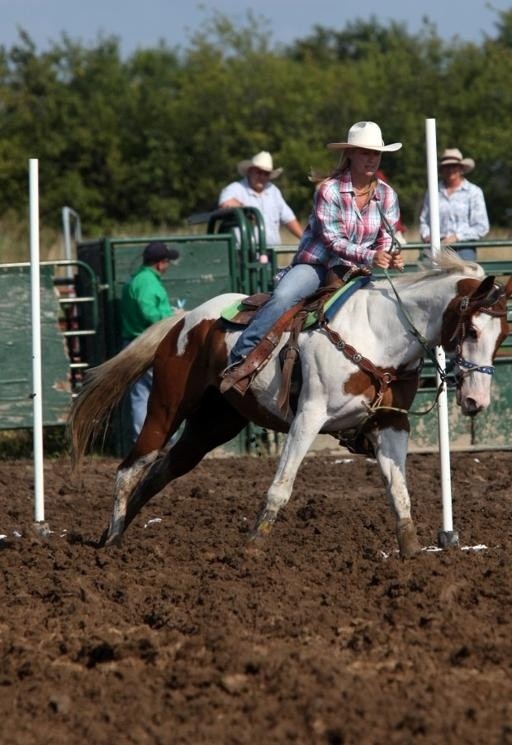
[62,247,512,563]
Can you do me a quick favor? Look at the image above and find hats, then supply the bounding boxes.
[239,150,284,180]
[143,242,180,267]
[439,147,476,174]
[327,121,403,153]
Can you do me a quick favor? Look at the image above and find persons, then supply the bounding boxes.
[221,119,407,399]
[118,238,188,465]
[417,147,492,263]
[216,149,304,273]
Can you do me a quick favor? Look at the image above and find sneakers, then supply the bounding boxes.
[225,360,250,392]
